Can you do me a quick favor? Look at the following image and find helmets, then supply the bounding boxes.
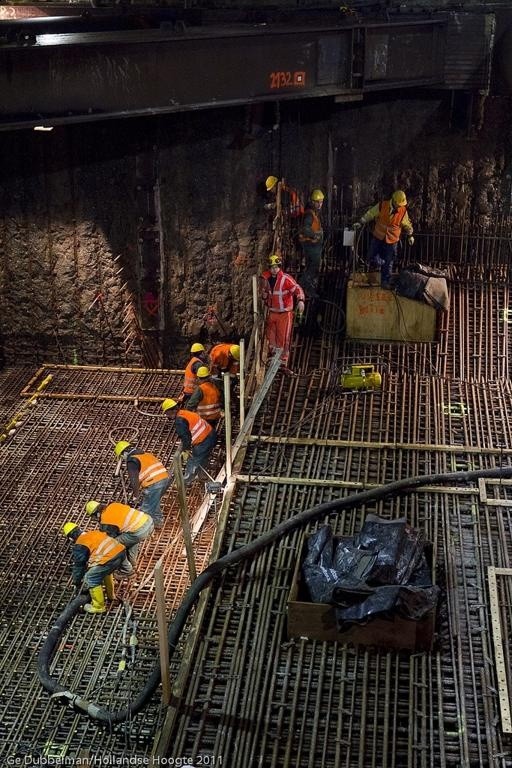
[162,398,177,414]
[265,176,279,191]
[392,190,408,206]
[115,441,131,456]
[197,366,210,378]
[63,522,78,536]
[267,255,282,266]
[311,189,324,201]
[190,343,205,352]
[230,345,240,360]
[86,500,101,516]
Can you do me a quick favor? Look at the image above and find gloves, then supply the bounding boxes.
[353,222,361,230]
[408,236,415,246]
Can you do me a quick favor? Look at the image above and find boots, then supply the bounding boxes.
[83,584,106,613]
[105,573,115,600]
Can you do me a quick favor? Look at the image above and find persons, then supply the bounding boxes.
[260,255,305,369]
[265,175,306,274]
[211,344,240,393]
[298,189,324,299]
[115,441,173,528]
[64,522,125,614]
[352,190,414,278]
[184,366,220,428]
[86,501,154,580]
[162,399,217,489]
[185,342,208,398]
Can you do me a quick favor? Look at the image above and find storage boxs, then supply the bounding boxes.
[283,531,441,655]
[344,270,437,344]
